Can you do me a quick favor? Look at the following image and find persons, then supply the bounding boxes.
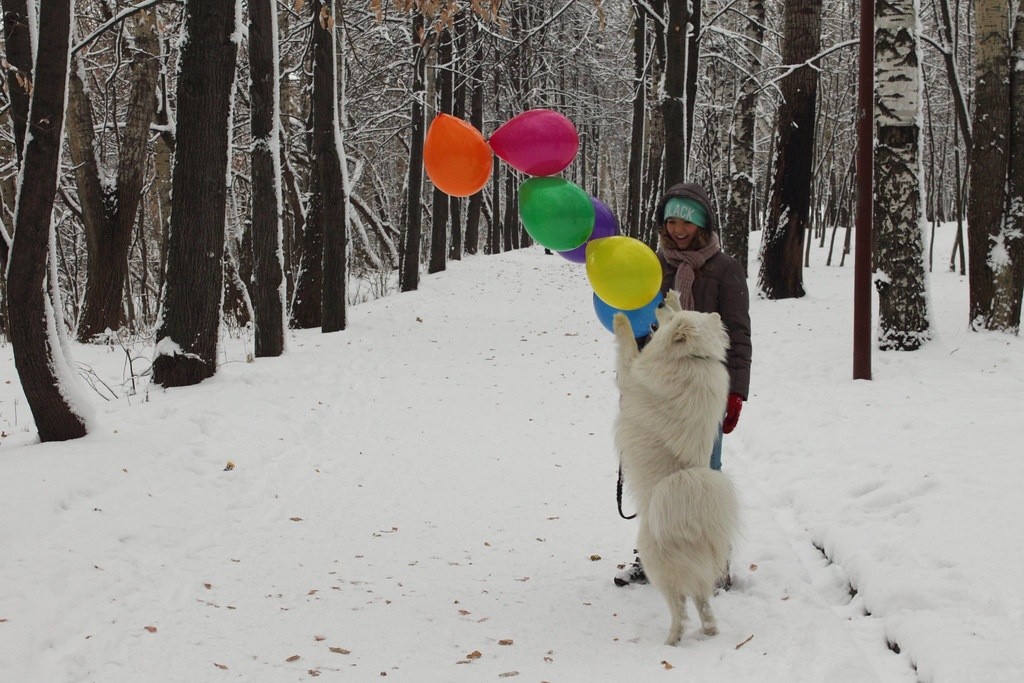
[614,184,752,593]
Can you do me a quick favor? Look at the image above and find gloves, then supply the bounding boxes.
[723,393,744,433]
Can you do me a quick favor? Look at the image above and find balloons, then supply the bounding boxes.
[424,114,493,197]
[518,177,595,253]
[558,197,618,264]
[488,109,579,179]
[585,236,663,311]
[593,290,663,338]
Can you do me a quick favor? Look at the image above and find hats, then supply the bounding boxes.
[666,197,708,228]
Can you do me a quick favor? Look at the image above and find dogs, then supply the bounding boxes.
[611,288,742,647]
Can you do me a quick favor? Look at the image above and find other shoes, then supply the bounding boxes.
[611,557,650,587]
